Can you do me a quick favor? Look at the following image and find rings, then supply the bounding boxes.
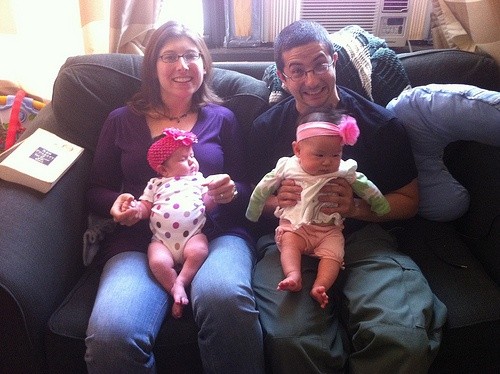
[220,193,224,200]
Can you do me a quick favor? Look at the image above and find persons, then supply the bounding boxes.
[84,19,265,374]
[245,109,393,309]
[245,20,448,374]
[118,126,218,319]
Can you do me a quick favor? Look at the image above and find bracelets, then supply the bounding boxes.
[231,185,238,199]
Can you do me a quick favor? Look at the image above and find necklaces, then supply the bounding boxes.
[156,109,191,123]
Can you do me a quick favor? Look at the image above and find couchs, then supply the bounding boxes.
[0,49,500,374]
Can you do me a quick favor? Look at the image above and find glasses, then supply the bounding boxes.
[282,61,334,81]
[157,52,200,63]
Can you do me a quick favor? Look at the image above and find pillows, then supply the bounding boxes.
[385,81,500,222]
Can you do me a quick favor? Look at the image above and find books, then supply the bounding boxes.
[0,127,85,193]
